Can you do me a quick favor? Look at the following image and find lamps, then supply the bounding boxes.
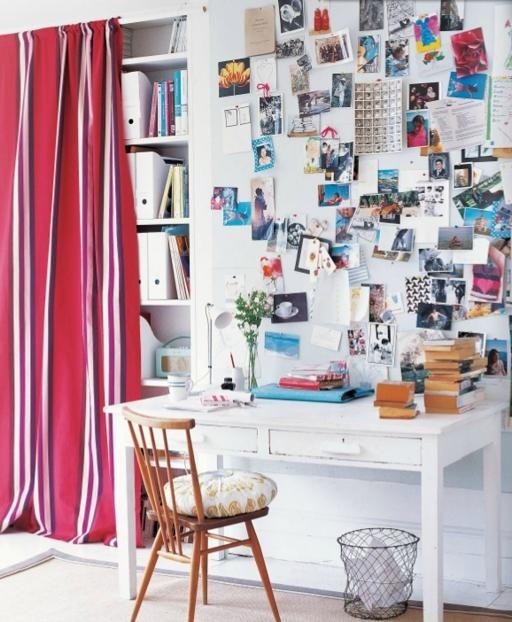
[204,303,231,384]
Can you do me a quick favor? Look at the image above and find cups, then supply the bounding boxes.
[276,301,293,315]
[167,374,194,398]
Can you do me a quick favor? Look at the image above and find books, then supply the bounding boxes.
[423,338,489,413]
[142,15,195,303]
[279,371,345,392]
[373,379,418,419]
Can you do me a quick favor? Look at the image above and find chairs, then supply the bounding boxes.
[121,406,281,622]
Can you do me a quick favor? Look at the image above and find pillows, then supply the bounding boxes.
[161,470,276,518]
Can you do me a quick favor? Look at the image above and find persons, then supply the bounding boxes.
[366,188,443,214]
[433,160,446,176]
[349,330,392,362]
[386,45,406,76]
[293,69,346,113]
[277,38,343,64]
[424,305,465,326]
[324,143,352,204]
[252,188,269,232]
[336,207,355,241]
[487,350,505,375]
[259,149,270,165]
[423,236,510,303]
[336,253,348,267]
[260,109,273,135]
[406,83,435,146]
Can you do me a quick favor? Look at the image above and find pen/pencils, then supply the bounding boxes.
[230,353,235,368]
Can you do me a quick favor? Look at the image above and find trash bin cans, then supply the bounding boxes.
[338,528,419,620]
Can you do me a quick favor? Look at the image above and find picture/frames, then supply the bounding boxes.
[295,235,332,275]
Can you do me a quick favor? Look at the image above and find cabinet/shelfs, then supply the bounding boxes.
[119,13,190,387]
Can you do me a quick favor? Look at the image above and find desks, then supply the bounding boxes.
[103,390,510,622]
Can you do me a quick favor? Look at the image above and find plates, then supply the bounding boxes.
[274,306,299,320]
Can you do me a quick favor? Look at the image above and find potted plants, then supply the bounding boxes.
[234,290,273,391]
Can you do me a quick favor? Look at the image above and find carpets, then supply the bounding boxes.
[0,548,512,622]
